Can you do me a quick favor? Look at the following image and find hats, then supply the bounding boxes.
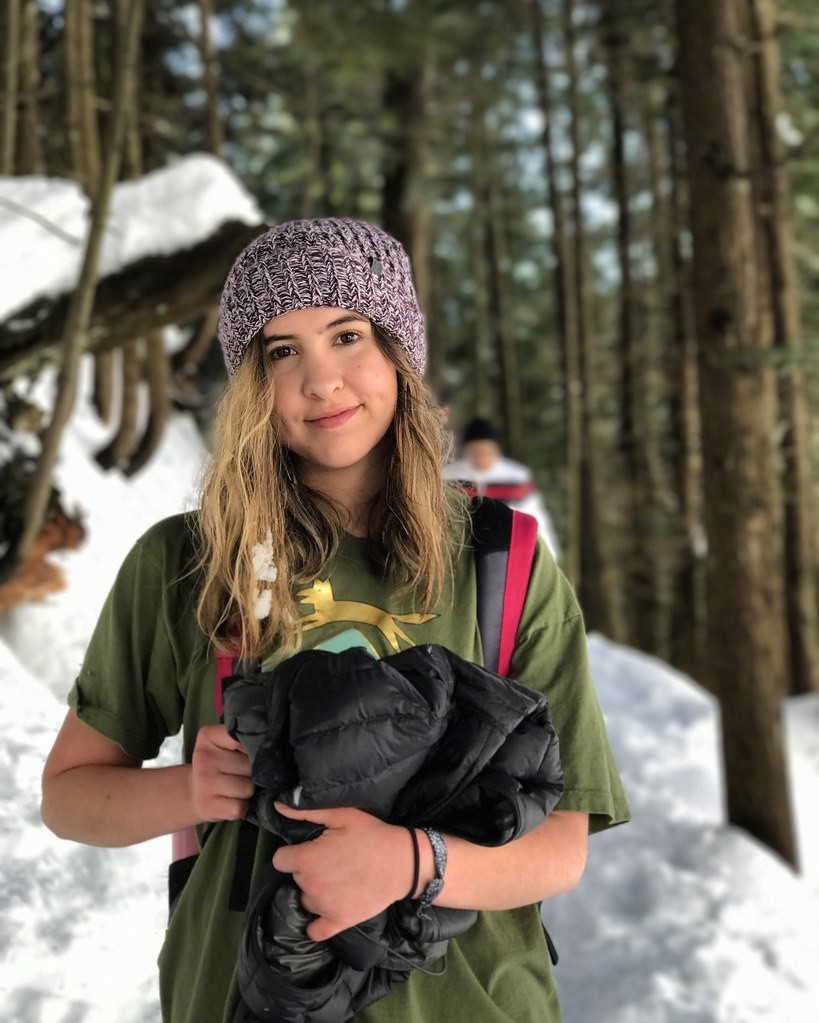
[463,417,496,441]
[219,217,427,385]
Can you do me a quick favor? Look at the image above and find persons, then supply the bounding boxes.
[441,418,558,562]
[40,218,630,1023]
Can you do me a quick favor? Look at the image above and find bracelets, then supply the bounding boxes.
[416,827,448,920]
[404,827,420,899]
